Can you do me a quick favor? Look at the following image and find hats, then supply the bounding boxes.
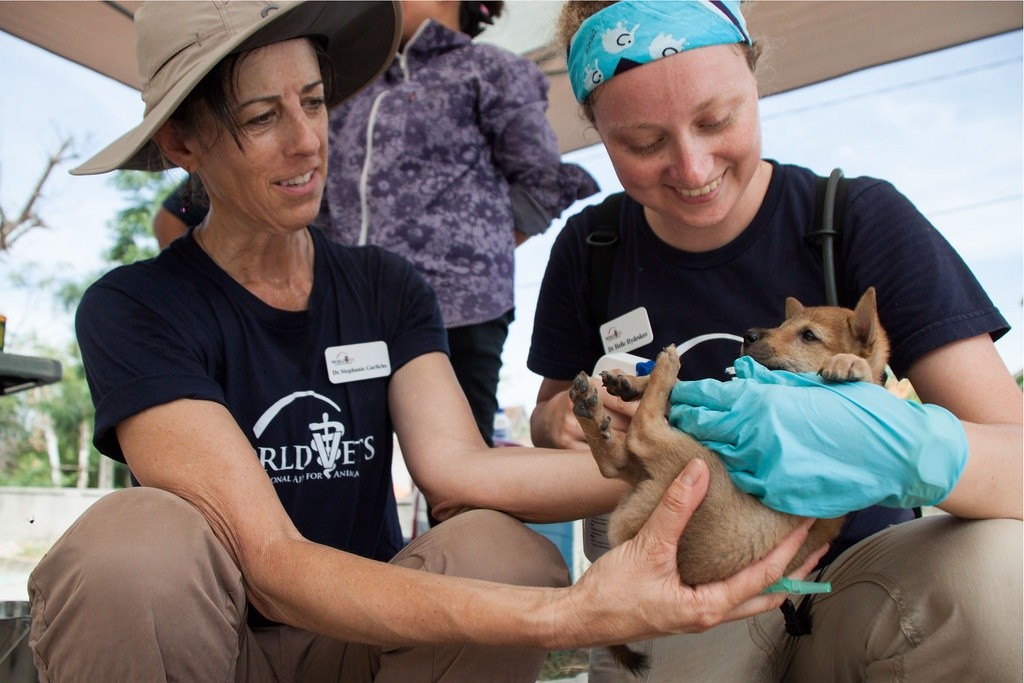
[68,0,404,174]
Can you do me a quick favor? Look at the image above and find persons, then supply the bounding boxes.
[26,0,831,683]
[526,0,1023,683]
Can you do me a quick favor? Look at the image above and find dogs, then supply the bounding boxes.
[568,286,891,677]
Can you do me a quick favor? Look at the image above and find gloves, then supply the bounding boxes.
[669,356,968,519]
